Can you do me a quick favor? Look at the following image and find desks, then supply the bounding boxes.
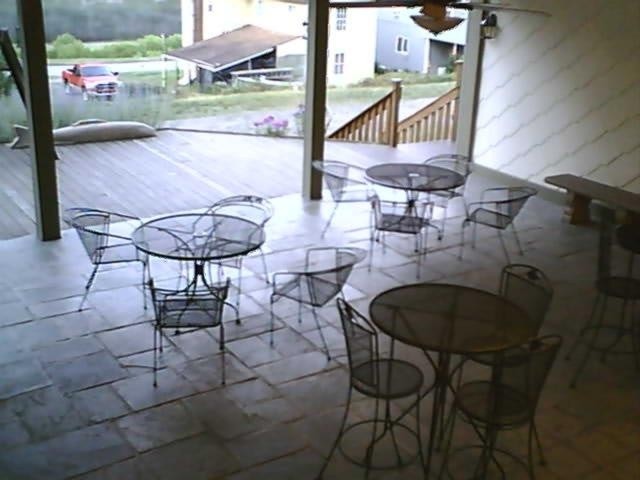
[368,282,542,480]
[130,210,267,337]
[362,161,469,252]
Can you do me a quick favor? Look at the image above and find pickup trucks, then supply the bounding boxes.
[62,62,125,100]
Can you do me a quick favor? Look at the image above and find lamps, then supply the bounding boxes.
[477,14,501,42]
[407,7,460,37]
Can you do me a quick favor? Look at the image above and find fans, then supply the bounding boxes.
[309,1,553,19]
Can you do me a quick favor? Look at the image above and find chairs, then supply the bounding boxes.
[459,183,539,266]
[436,332,564,479]
[266,244,368,363]
[312,157,382,240]
[190,193,275,305]
[416,153,474,238]
[566,208,640,389]
[145,275,234,389]
[366,192,429,282]
[443,262,555,428]
[61,206,152,312]
[319,295,427,479]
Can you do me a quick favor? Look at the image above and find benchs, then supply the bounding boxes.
[544,169,639,230]
